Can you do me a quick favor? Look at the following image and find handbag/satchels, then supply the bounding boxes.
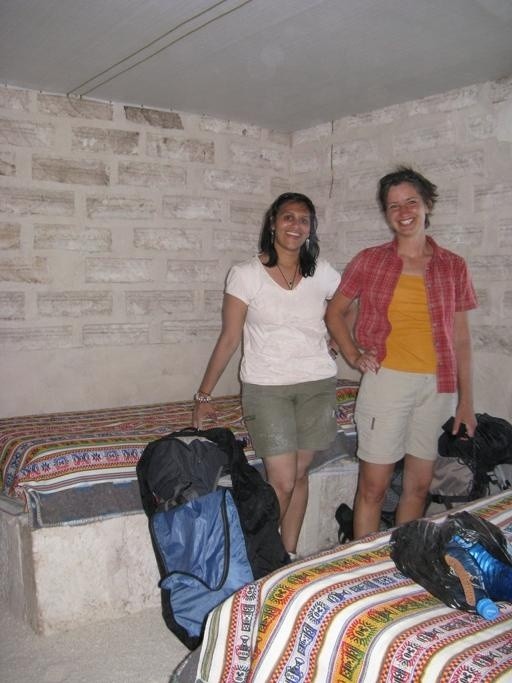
[437,411,512,467]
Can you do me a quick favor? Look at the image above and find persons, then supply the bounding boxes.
[191,191,359,561]
[324,165,479,542]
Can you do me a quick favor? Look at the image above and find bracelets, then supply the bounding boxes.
[326,341,339,357]
[194,391,212,403]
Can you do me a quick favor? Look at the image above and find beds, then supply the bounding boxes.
[176,490,512,682]
[1,378,363,636]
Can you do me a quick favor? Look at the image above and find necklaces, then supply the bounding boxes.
[275,262,298,289]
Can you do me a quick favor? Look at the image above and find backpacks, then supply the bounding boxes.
[136,426,292,652]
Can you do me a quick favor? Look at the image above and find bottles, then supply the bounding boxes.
[445,523,512,620]
